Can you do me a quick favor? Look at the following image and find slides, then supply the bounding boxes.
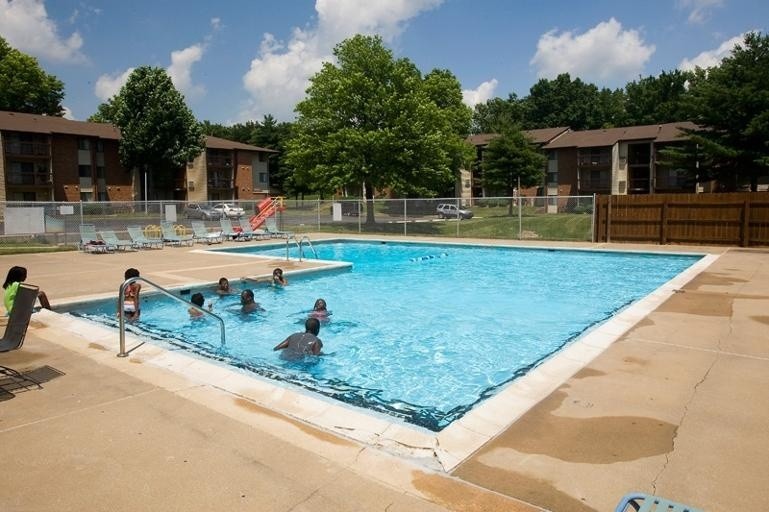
[232,197,273,233]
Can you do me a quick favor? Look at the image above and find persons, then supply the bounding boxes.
[309,299,330,322]
[274,318,322,356]
[3,266,36,316]
[258,269,286,287]
[117,268,140,322]
[241,290,257,313]
[216,278,235,295]
[189,293,212,315]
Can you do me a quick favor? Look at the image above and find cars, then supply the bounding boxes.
[182,202,220,221]
[330,201,363,217]
[212,203,245,220]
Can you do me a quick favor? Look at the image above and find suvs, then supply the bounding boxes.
[436,204,473,220]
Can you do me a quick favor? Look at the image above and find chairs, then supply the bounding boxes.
[0,281,44,397]
[77,217,296,255]
